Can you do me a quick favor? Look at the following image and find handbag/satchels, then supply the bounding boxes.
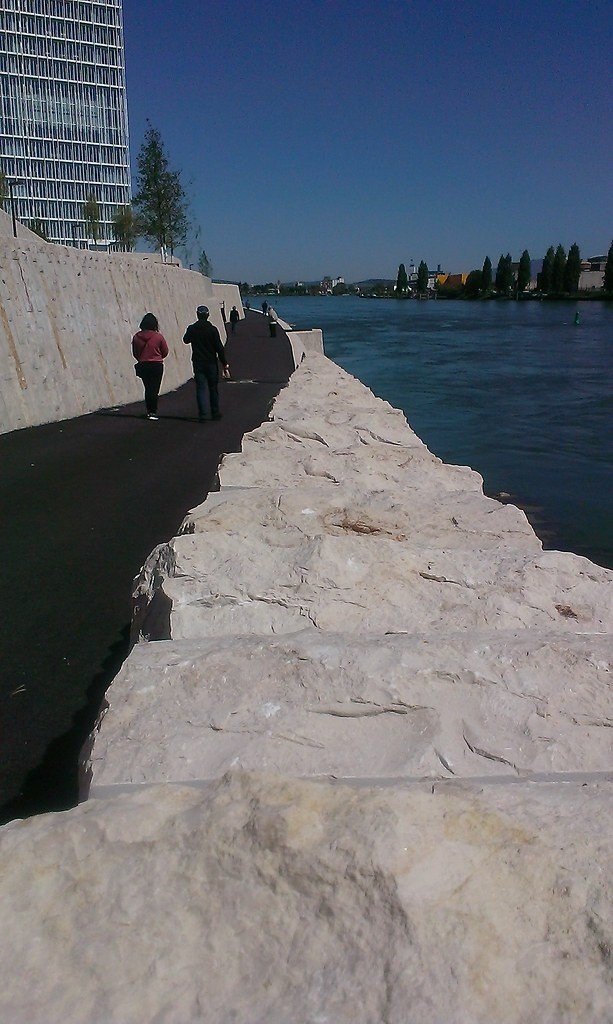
[134,364,141,376]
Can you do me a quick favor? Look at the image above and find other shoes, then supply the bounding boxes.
[199,419,208,423]
[213,414,222,421]
[147,413,159,420]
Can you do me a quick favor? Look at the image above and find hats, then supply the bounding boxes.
[196,306,209,314]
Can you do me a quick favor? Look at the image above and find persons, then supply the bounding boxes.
[246,299,250,311]
[261,301,268,316]
[230,306,239,333]
[182,305,229,422]
[268,306,278,337]
[131,312,169,420]
